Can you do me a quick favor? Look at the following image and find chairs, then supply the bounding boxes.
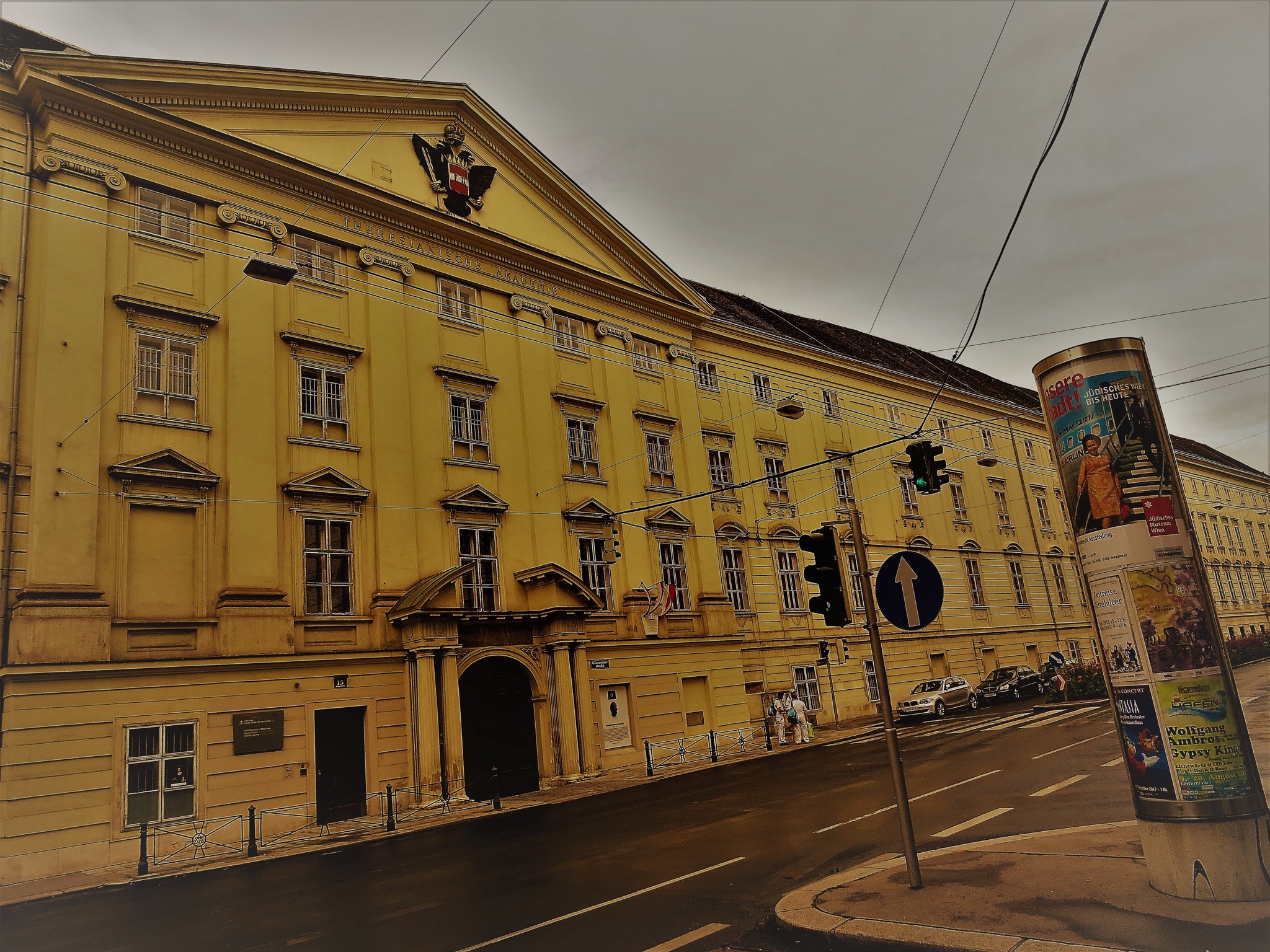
[927,682,942,692]
[998,672,1014,678]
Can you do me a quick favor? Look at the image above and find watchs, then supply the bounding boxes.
[1116,485,1122,487]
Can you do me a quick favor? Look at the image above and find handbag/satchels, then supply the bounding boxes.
[806,720,815,738]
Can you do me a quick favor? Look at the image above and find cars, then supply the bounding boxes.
[895,674,978,722]
[1038,657,1081,689]
[974,664,1044,706]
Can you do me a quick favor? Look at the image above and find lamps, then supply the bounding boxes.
[243,252,299,286]
[776,399,805,420]
[976,454,998,467]
[1258,510,1268,516]
[1212,501,1224,511]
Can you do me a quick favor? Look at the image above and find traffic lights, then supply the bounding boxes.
[799,524,852,627]
[836,638,850,664]
[905,441,934,492]
[924,443,950,493]
[818,640,830,658]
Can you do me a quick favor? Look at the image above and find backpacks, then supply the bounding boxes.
[768,699,782,717]
[787,701,798,725]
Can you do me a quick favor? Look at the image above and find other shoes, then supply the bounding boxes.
[781,742,790,745]
[779,743,782,746]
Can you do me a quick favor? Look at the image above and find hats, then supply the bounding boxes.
[790,688,795,693]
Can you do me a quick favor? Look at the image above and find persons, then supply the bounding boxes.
[1104,642,1140,674]
[1077,434,1132,530]
[784,688,805,742]
[773,691,790,746]
[787,693,810,745]
[1126,387,1171,489]
